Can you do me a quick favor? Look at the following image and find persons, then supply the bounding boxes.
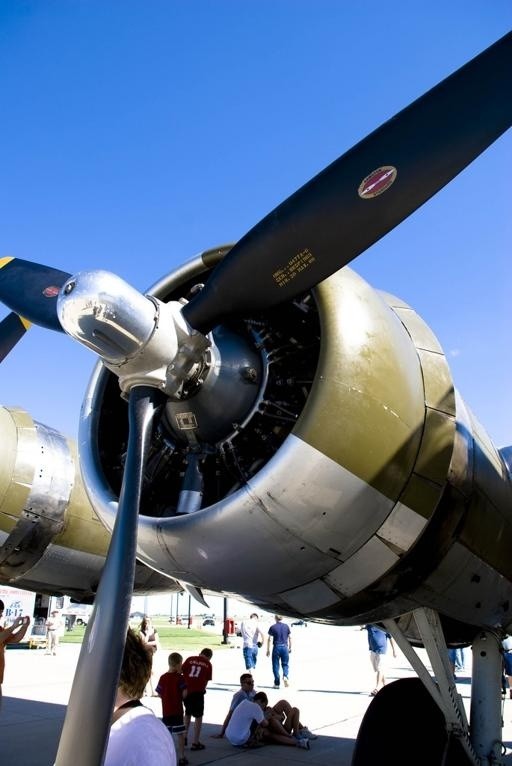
[155,651,190,766]
[45,607,61,654]
[241,612,265,679]
[502,635,512,700]
[266,614,292,689]
[135,616,162,697]
[359,622,399,697]
[250,696,307,741]
[210,671,318,750]
[447,646,466,680]
[101,623,178,766]
[224,691,270,749]
[0,598,31,688]
[181,647,214,751]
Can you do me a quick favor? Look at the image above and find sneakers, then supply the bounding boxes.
[191,742,205,751]
[273,676,288,689]
[179,757,188,765]
[184,738,188,745]
[293,727,317,749]
[371,688,378,695]
